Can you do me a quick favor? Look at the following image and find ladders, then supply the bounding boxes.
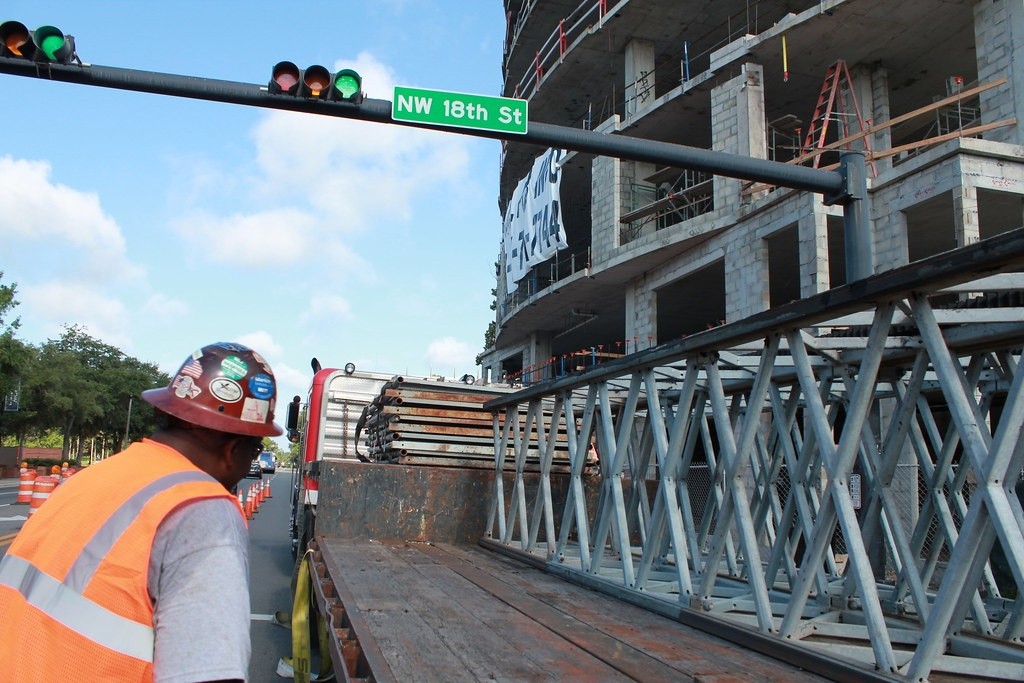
[797,57,879,180]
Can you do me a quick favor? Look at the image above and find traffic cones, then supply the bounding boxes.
[237,477,274,520]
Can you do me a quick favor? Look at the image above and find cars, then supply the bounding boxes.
[245,452,277,479]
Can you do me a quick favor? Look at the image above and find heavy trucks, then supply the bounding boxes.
[285,355,1024,683]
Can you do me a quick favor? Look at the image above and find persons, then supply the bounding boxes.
[2,343,280,683]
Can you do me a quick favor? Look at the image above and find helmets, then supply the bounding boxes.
[141,342,284,438]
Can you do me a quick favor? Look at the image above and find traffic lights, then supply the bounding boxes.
[1,20,77,65]
[268,60,364,104]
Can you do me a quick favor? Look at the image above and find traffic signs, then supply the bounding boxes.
[390,85,528,135]
